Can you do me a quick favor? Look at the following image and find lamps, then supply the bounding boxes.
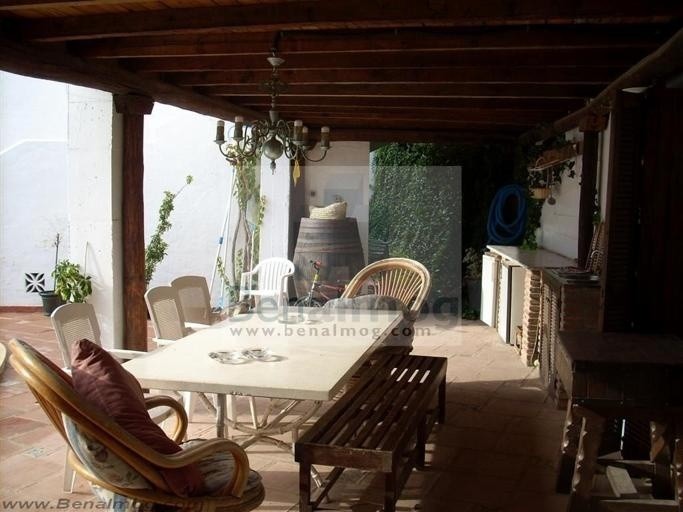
[213,31,332,175]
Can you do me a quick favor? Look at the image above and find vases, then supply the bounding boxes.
[39,291,65,315]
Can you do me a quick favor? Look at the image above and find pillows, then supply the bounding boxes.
[70,339,204,498]
[321,295,414,352]
[60,412,153,491]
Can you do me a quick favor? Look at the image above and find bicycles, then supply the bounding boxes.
[294,256,380,309]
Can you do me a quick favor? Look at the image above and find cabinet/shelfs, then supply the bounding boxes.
[479,244,582,346]
[538,268,604,410]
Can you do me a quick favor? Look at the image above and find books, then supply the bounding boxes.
[552,268,590,277]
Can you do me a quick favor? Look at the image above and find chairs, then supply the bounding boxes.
[144,286,236,438]
[238,256,295,323]
[169,276,258,430]
[340,257,431,403]
[0,339,265,512]
[50,303,186,494]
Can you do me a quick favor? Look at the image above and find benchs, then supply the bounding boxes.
[294,352,448,511]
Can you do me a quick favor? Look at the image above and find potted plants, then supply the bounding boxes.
[463,247,486,312]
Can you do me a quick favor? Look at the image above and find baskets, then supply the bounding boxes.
[528,184,552,200]
[308,201,348,220]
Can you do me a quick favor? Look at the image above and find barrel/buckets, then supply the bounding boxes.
[292,216,365,307]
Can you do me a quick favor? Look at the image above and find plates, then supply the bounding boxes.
[208,346,271,365]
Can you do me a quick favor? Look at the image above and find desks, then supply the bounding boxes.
[553,330,683,512]
[119,304,404,503]
